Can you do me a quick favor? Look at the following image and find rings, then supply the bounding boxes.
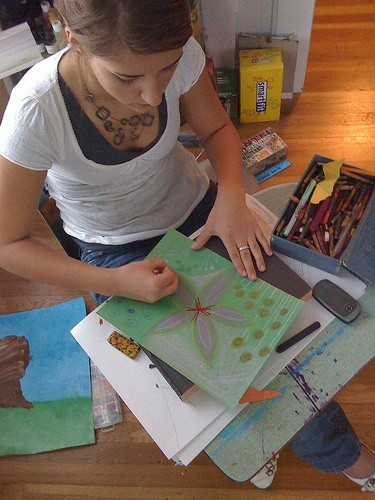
[239,245,249,251]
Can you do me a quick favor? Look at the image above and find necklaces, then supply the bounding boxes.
[78,54,154,146]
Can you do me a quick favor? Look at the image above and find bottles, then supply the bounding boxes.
[0,0,69,55]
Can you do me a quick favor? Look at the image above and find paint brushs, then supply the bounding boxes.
[274,320,322,354]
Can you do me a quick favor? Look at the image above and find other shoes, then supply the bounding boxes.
[249,452,279,488]
[341,439,375,494]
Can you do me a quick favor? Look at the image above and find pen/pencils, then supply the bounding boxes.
[271,154,375,261]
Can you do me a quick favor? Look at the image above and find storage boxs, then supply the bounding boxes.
[272,154,375,286]
[240,48,283,121]
[241,128,288,175]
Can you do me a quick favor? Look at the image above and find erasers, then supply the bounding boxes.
[106,330,141,360]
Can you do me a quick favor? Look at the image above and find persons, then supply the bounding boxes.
[0,0,375,492]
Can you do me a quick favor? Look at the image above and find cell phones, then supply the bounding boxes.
[312,278,363,325]
